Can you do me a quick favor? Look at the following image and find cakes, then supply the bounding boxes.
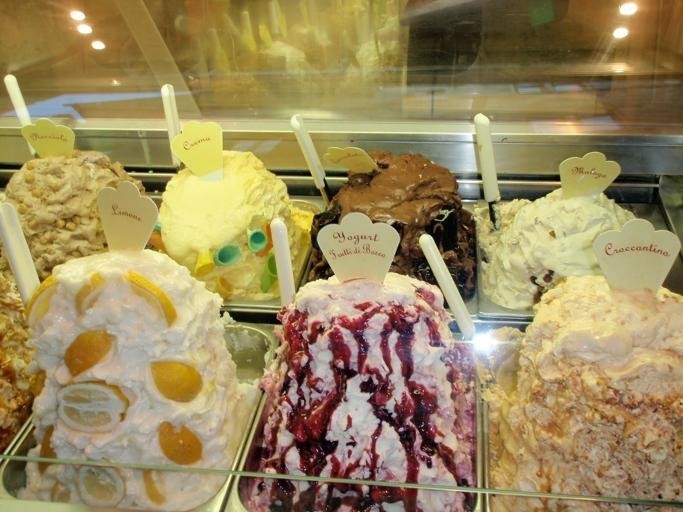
[481,186,638,309]
[159,147,305,303]
[188,0,400,117]
[311,148,476,308]
[1,272,39,445]
[489,273,679,511]
[30,255,237,510]
[1,147,156,272]
[248,272,477,511]
